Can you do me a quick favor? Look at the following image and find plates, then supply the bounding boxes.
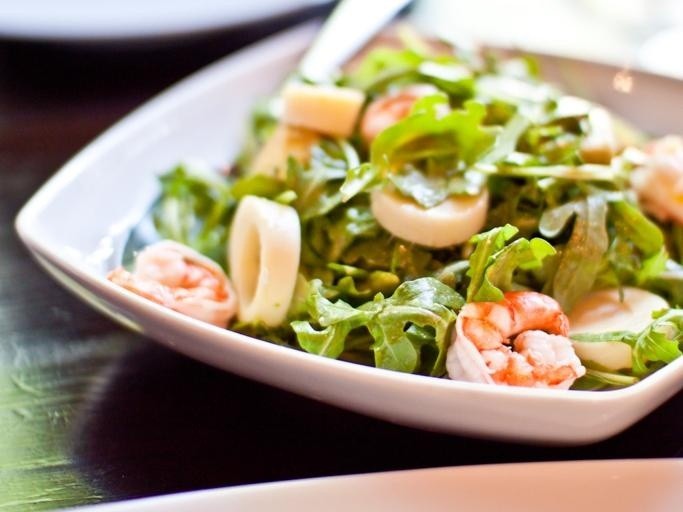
[13,24,683,445]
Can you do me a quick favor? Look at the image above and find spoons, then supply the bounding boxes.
[121,0,414,277]
